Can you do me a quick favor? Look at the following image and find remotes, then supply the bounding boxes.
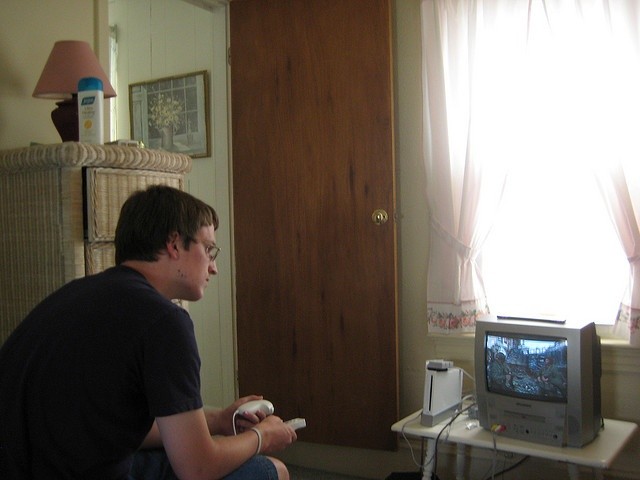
[271,415,308,441]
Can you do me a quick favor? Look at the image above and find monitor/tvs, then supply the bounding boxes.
[474,314,602,448]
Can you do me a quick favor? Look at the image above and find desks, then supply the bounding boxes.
[392,395,638,479]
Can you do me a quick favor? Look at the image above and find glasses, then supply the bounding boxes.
[178,228,223,262]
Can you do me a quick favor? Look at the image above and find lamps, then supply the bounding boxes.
[32,41,116,142]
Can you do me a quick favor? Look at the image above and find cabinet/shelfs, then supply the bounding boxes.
[2,147,194,341]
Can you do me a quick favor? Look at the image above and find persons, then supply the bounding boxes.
[0,183,297,480]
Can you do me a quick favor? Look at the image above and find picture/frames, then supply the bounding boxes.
[129,71,211,158]
